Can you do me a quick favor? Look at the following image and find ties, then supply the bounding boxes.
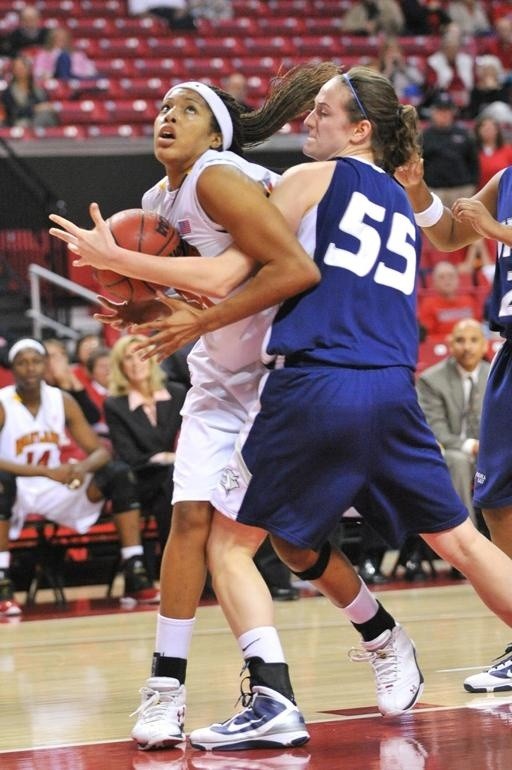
[467,376,475,438]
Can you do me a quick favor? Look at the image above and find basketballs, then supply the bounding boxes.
[89,207,181,301]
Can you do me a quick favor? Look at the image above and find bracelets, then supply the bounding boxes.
[412,191,444,229]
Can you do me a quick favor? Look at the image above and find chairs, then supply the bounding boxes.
[2,217,505,378]
[0,0,510,145]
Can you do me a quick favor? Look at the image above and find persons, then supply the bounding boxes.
[48,63,511,750]
[1,0,101,126]
[391,146,512,695]
[414,318,491,577]
[130,1,235,33]
[88,65,422,752]
[0,321,301,616]
[342,0,512,197]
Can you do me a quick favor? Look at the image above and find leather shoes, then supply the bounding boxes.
[403,558,426,581]
[270,586,298,601]
[359,555,386,584]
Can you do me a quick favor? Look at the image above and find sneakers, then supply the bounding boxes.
[123,572,160,604]
[1,578,24,616]
[347,621,423,720]
[130,675,186,750]
[463,645,512,694]
[189,674,311,752]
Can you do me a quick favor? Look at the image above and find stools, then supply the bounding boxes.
[10,503,468,616]
